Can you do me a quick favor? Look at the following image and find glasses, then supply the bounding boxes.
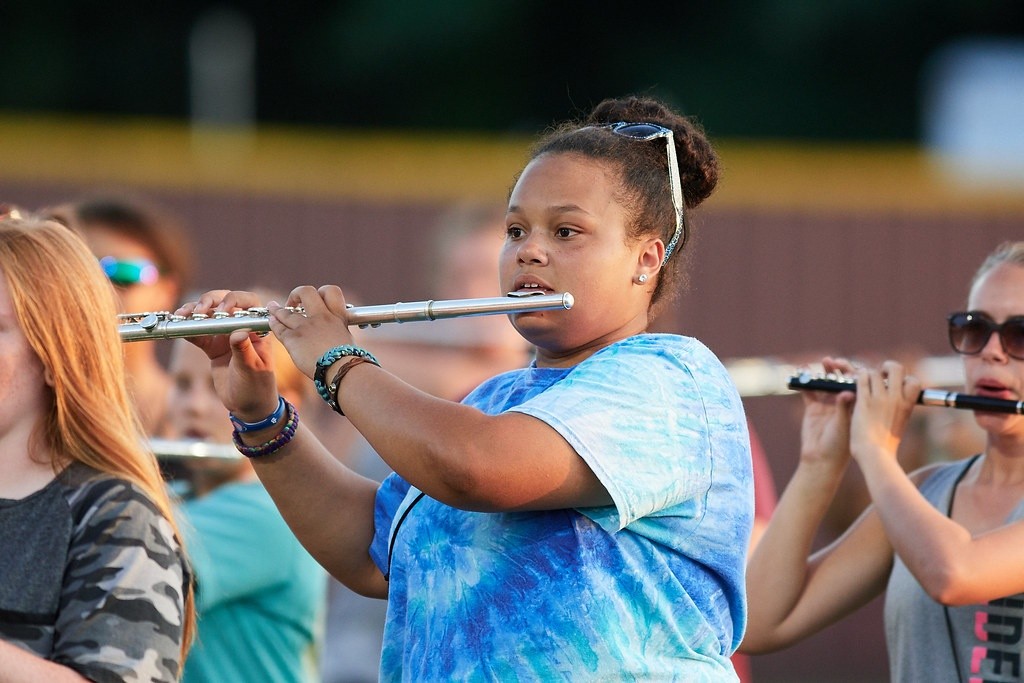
[98,258,173,289]
[945,310,1024,360]
[597,122,683,267]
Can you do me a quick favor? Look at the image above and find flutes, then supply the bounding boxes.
[784,364,1024,416]
[116,290,576,345]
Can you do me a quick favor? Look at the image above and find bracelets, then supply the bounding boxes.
[232,398,300,458]
[327,357,382,417]
[313,345,377,403]
[229,396,285,433]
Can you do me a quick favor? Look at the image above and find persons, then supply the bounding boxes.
[731,422,776,683]
[737,242,1024,683]
[0,197,332,683]
[172,96,756,682]
